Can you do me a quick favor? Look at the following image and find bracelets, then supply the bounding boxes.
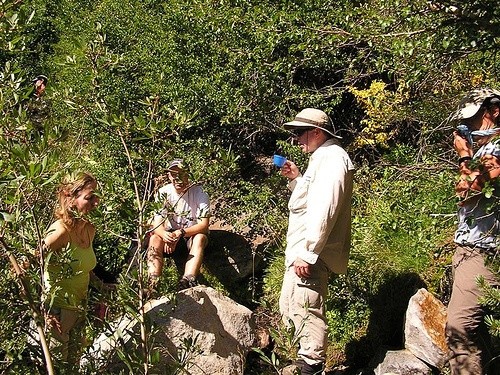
[179,228,186,238]
[458,156,472,167]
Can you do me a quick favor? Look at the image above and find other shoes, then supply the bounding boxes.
[176,275,198,292]
[281,359,326,375]
[142,282,161,299]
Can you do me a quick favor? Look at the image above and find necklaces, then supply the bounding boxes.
[74,229,85,244]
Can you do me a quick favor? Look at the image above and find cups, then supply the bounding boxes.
[274,155,287,167]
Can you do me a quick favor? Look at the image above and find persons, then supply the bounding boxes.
[444,87,500,375]
[10,173,101,375]
[278,108,354,375]
[136,159,210,299]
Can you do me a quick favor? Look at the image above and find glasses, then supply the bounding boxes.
[289,127,317,138]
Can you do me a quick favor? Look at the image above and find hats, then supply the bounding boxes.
[458,87,500,119]
[168,159,190,174]
[283,108,343,139]
